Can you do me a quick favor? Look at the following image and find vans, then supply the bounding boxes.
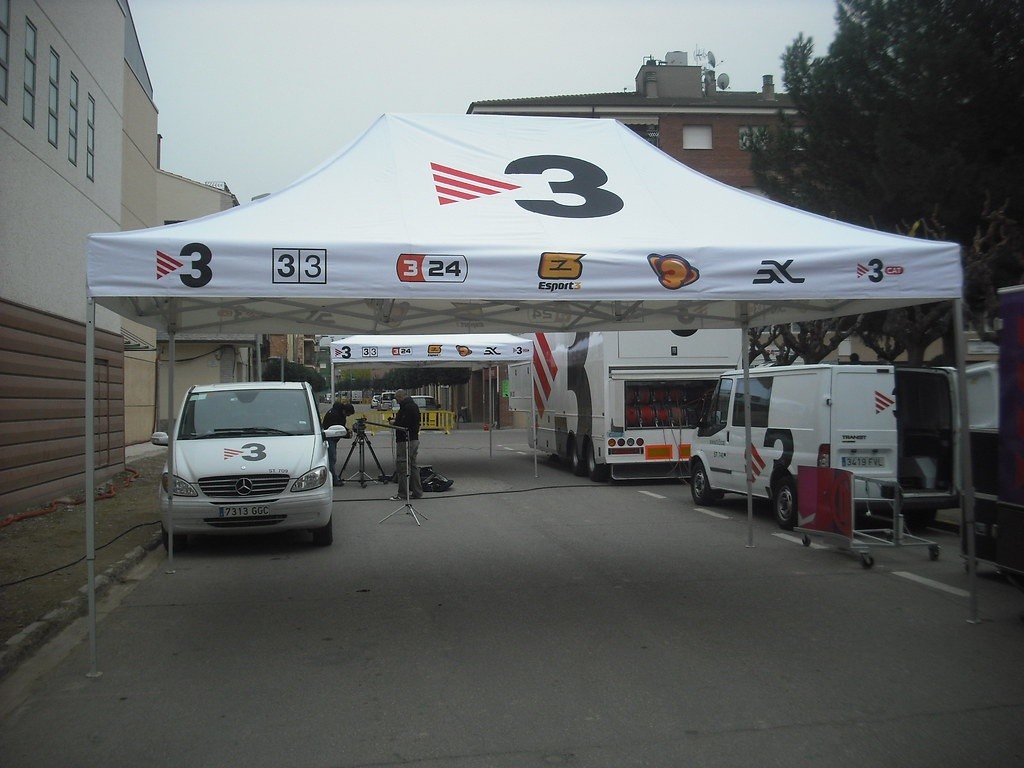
[687,361,1000,530]
[392,394,441,413]
[318,390,396,411]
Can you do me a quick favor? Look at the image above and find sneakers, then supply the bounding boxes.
[389,495,407,501]
[409,493,419,499]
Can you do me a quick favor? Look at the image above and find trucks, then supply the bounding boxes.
[506,322,744,484]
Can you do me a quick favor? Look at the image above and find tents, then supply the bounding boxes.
[329,332,538,484]
[84,116,980,682]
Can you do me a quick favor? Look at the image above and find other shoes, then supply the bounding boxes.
[333,480,344,486]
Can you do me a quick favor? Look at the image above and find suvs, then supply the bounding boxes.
[150,380,350,556]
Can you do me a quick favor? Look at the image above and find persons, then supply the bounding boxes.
[321,399,355,486]
[391,388,423,499]
